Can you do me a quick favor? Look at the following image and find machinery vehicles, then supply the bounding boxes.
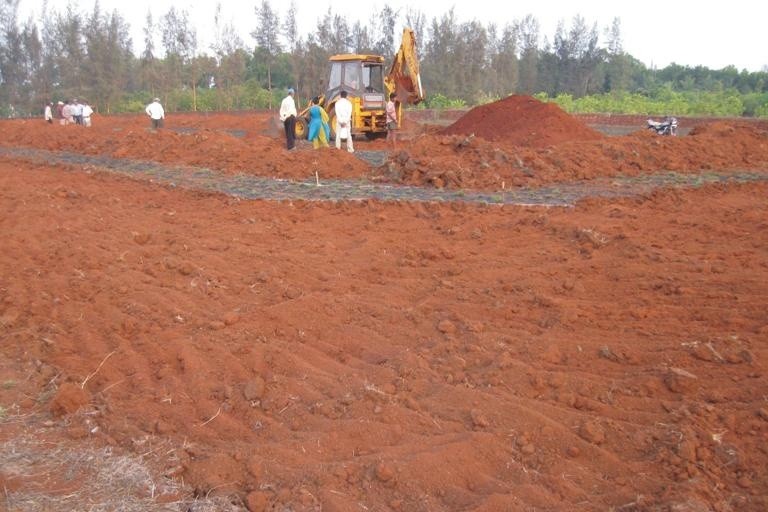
[291,28,425,140]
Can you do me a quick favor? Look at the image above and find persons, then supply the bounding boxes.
[57,100,66,125]
[145,97,166,129]
[279,87,298,152]
[386,92,399,144]
[71,98,84,126]
[81,100,94,128]
[335,90,355,154]
[62,100,75,125]
[44,102,55,124]
[306,96,330,151]
